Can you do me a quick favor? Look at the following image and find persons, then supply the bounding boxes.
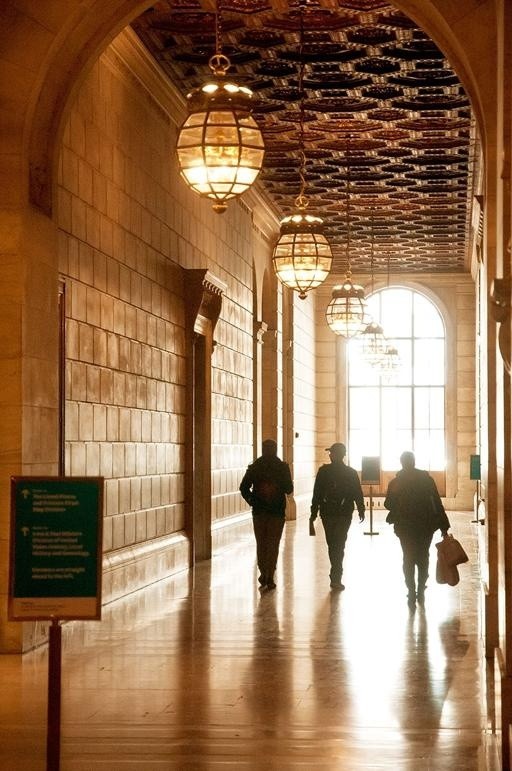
[238,439,295,594]
[383,452,452,612]
[307,441,366,593]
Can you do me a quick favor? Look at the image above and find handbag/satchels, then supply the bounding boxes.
[436,534,470,566]
[435,552,460,587]
[426,494,442,532]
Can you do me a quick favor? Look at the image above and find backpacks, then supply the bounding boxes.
[319,464,351,520]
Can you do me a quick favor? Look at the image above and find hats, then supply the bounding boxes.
[323,442,347,453]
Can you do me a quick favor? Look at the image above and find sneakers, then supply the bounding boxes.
[258,574,279,591]
[406,591,416,602]
[329,579,346,592]
[417,587,426,600]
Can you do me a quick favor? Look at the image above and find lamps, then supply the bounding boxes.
[166,0,269,213]
[325,130,403,372]
[272,0,331,305]
